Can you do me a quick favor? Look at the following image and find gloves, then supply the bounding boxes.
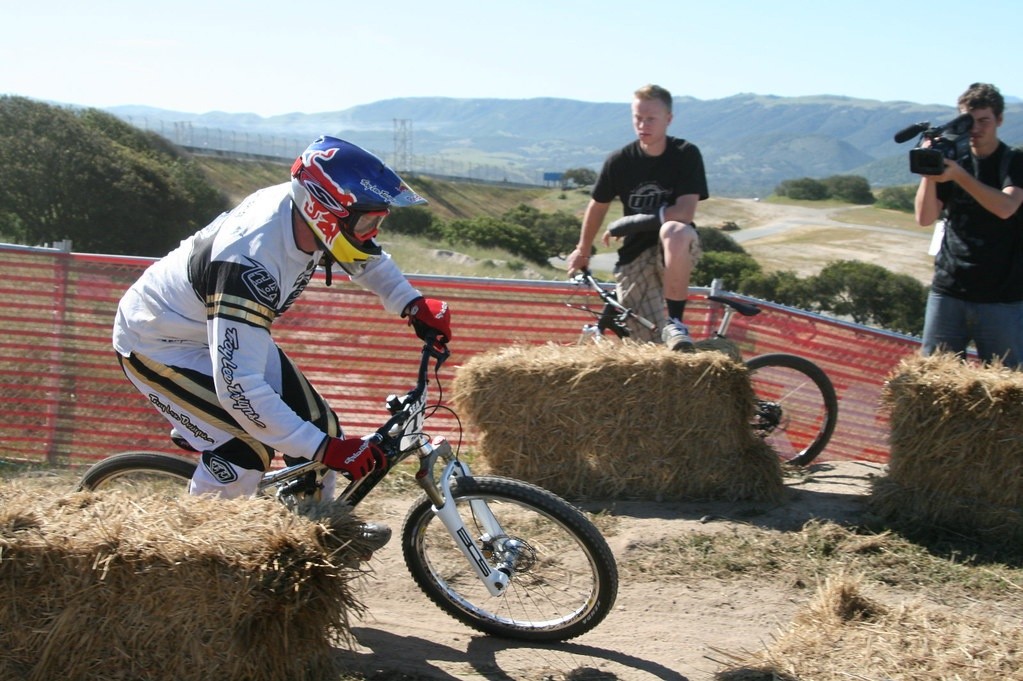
[322,436,387,481]
[407,299,452,351]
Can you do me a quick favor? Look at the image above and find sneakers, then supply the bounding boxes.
[661,319,692,351]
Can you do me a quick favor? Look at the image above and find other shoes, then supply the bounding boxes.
[341,521,392,550]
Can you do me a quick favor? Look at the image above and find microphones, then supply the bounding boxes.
[894,121,929,144]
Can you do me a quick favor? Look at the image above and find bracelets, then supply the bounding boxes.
[575,244,590,261]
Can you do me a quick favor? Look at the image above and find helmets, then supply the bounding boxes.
[289,134,429,276]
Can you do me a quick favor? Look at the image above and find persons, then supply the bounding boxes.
[912,82,1023,372]
[567,84,710,352]
[110,134,452,555]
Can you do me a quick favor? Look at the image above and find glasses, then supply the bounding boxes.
[354,210,391,242]
